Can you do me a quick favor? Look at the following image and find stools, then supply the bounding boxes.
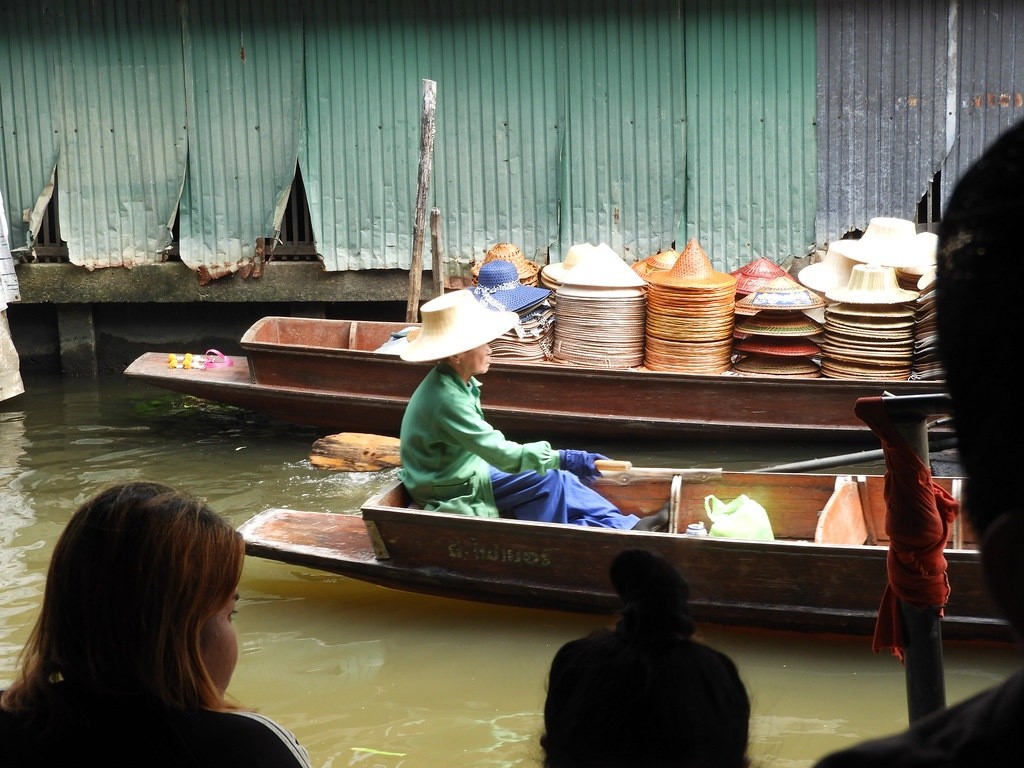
[402,497,511,519]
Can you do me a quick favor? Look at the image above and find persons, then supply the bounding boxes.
[400,290,669,532]
[0,483,313,768]
[540,551,751,768]
[812,120,1024,768]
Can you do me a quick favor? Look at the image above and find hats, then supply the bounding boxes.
[464,215,951,382]
[610,550,690,609]
[399,288,519,362]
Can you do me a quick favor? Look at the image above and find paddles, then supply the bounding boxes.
[306,431,636,475]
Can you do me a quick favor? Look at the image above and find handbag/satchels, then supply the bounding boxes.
[704,493,774,541]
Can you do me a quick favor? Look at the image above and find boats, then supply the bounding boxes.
[234,467,1017,644]
[124,315,956,443]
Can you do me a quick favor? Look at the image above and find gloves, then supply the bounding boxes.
[561,449,610,484]
